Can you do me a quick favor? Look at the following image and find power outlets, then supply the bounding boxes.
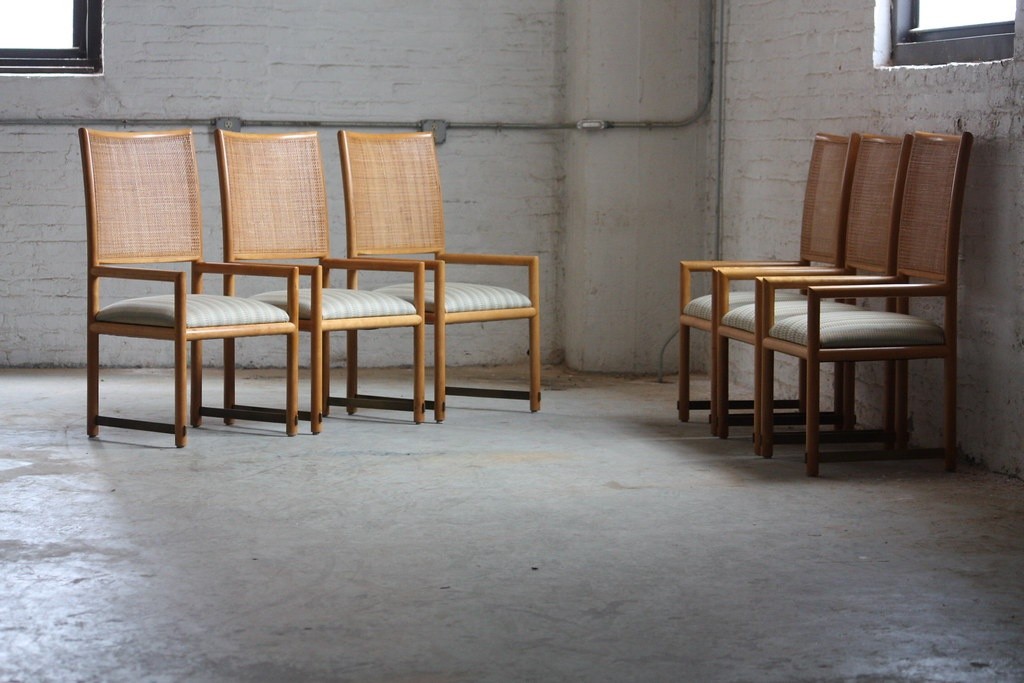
[420,119,446,145]
[216,116,241,133]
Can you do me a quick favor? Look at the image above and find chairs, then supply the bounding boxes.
[715,132,914,456]
[760,130,975,479]
[336,129,542,424]
[212,127,425,436]
[676,131,861,437]
[77,126,301,449]
